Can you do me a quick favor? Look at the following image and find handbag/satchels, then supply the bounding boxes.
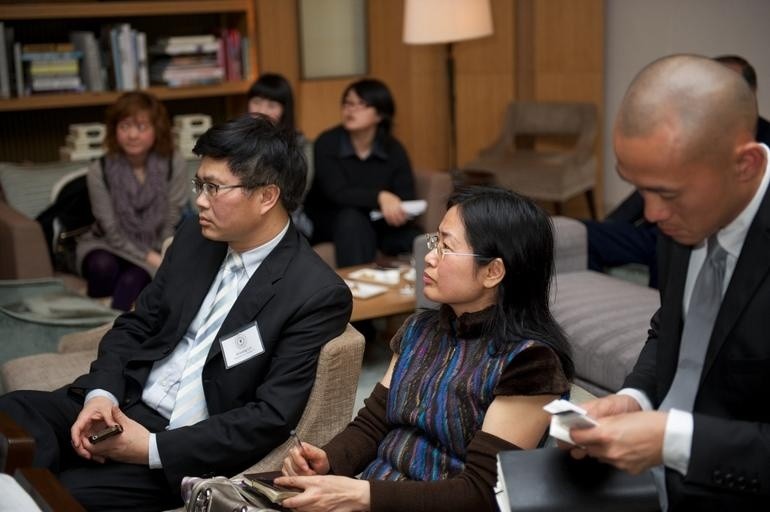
[55,217,102,273]
[181,476,286,512]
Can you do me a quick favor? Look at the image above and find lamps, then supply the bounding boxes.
[402,0,495,178]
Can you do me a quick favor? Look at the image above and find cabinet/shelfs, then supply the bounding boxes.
[0,0,260,163]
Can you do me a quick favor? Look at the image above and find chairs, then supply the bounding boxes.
[0,465,88,512]
[466,98,601,224]
[0,321,365,512]
[0,409,36,477]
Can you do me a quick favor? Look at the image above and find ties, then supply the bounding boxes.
[165,252,245,431]
[650,233,728,512]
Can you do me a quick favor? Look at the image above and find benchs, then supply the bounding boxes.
[413,214,661,398]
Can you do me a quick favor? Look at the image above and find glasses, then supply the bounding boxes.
[425,231,480,261]
[191,176,245,198]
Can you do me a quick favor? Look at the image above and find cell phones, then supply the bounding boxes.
[89,425,123,444]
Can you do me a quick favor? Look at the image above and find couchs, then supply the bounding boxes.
[0,141,454,303]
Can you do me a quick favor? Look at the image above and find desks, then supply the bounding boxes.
[334,258,419,355]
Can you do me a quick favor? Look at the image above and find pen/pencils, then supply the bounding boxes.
[290,430,314,470]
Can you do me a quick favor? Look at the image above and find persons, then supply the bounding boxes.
[273,184,576,512]
[76,92,191,312]
[557,53,770,512]
[579,56,770,289]
[303,79,425,365]
[244,74,315,242]
[0,112,353,512]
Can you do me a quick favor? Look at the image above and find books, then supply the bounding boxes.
[0,19,250,98]
[242,470,304,504]
